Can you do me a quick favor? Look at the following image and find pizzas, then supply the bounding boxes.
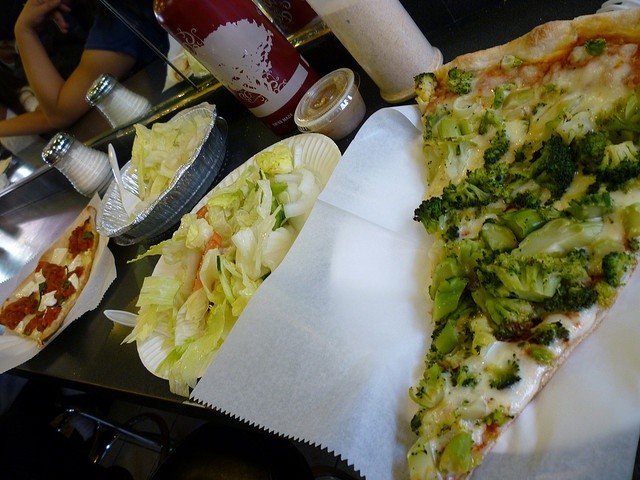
[399,10,637,476]
[1,206,94,341]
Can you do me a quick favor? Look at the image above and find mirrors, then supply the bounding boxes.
[0,0,211,200]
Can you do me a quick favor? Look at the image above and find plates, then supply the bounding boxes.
[136,134,342,381]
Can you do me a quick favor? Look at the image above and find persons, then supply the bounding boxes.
[1,0,170,138]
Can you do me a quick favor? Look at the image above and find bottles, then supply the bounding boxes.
[41,131,118,198]
[153,0,306,138]
[257,0,318,37]
[85,74,153,130]
[305,0,443,103]
